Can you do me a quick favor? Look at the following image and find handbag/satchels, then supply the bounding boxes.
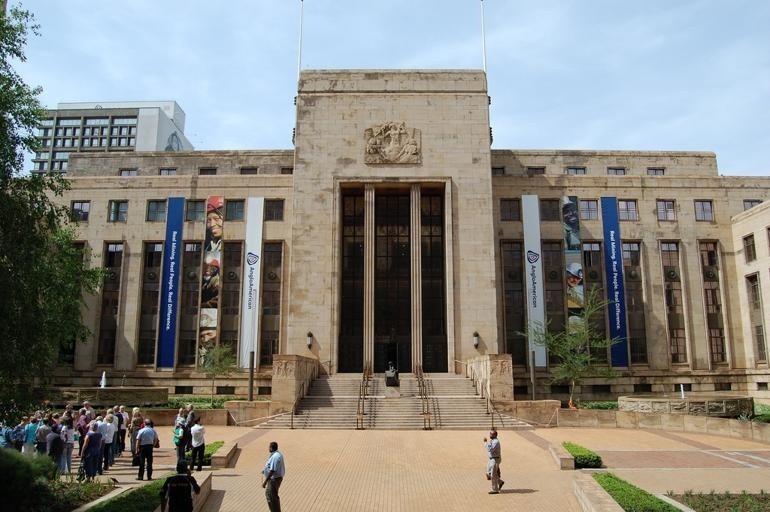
[76,466,85,481]
[132,454,140,466]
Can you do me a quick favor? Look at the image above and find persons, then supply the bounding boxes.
[159,460,200,512]
[566,262,584,308]
[261,442,285,512]
[484,430,504,494]
[199,313,217,369]
[562,202,581,251]
[204,203,224,251]
[200,258,221,308]
[0,399,205,480]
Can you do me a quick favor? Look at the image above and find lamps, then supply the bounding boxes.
[307,332,313,349]
[473,331,479,349]
